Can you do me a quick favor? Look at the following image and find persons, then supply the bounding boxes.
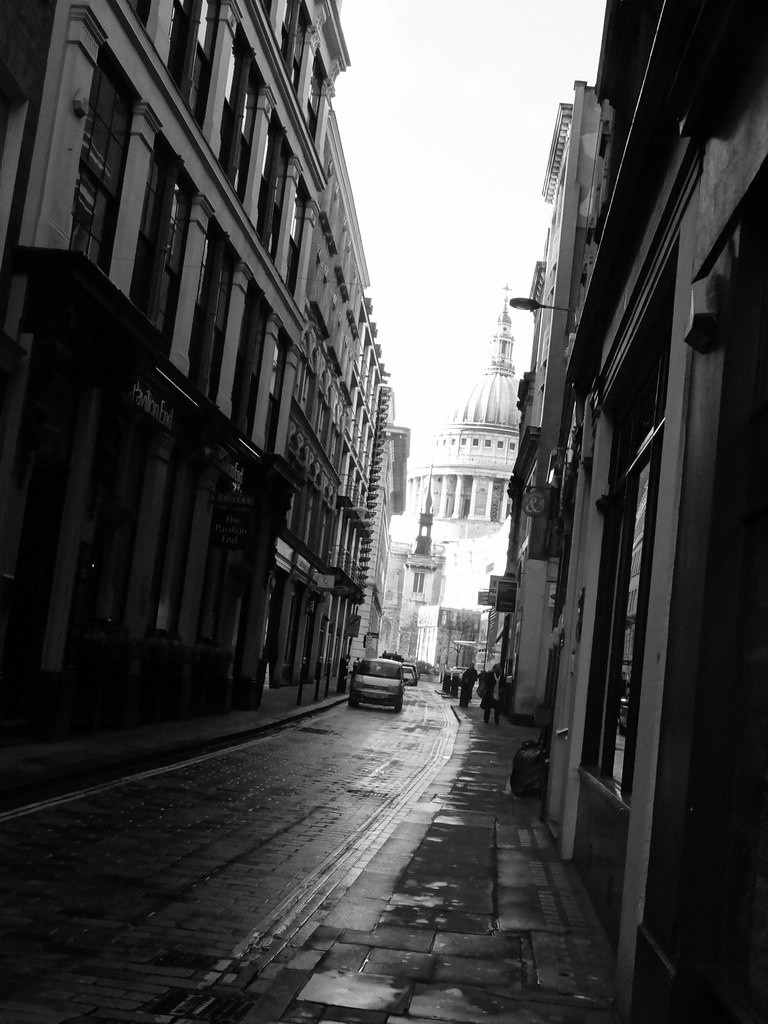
[459,663,487,707]
[484,664,506,726]
[353,657,360,672]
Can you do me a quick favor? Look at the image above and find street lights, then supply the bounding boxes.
[510,296,573,822]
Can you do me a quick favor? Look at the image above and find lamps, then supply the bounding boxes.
[509,298,576,314]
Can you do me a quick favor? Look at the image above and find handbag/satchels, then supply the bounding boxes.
[510,739,543,797]
[476,685,488,698]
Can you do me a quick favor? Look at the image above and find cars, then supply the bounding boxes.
[401,662,419,685]
[349,658,404,711]
